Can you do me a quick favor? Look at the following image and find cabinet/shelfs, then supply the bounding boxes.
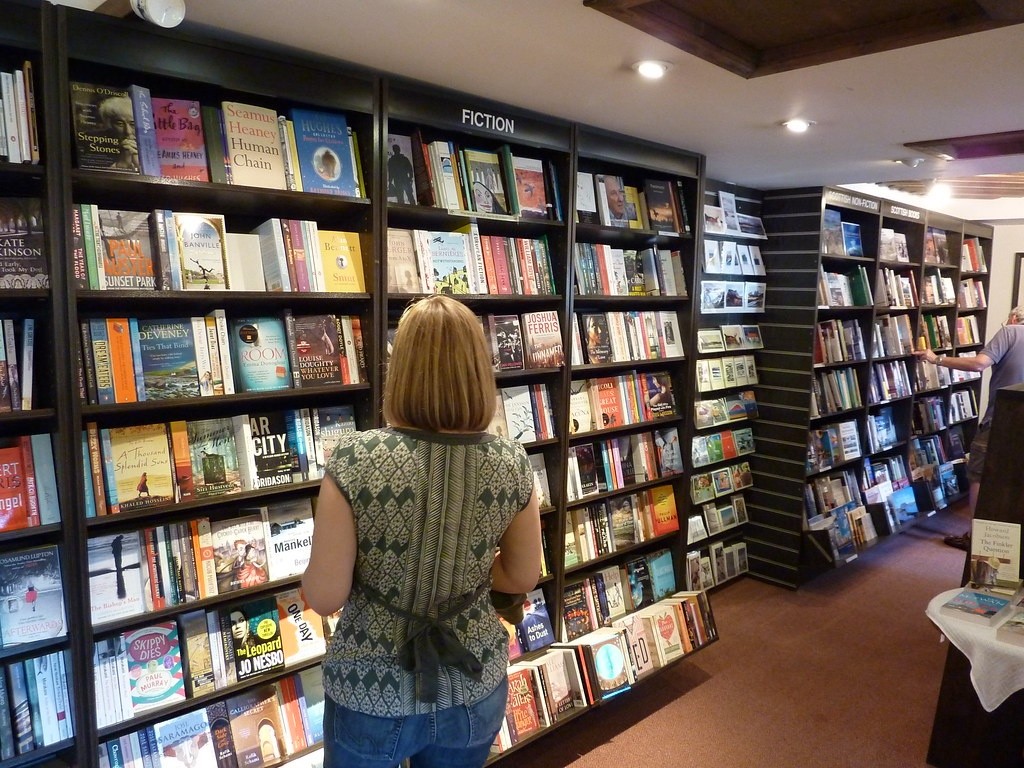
[866,195,931,557]
[50,4,383,766]
[903,205,966,534]
[376,66,592,768]
[687,175,776,616]
[761,187,884,590]
[0,1,96,768]
[560,115,711,745]
[946,216,997,514]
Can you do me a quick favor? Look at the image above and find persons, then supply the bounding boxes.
[300,296,542,768]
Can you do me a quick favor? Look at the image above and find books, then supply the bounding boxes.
[1,58,769,768]
[804,208,987,569]
[940,516,1024,650]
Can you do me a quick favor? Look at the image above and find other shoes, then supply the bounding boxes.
[945,532,970,551]
